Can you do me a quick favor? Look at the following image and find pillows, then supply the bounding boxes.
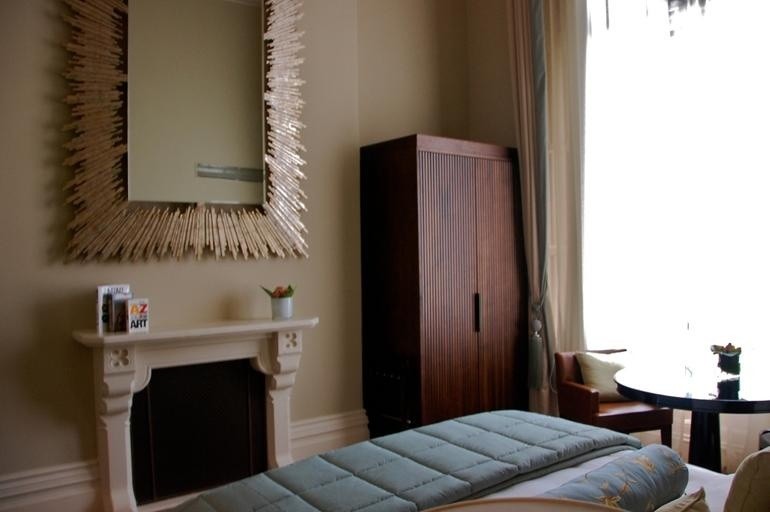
[576,351,630,400]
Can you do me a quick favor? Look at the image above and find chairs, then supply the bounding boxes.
[554,349,674,452]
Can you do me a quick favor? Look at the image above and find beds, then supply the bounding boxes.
[159,410,770,512]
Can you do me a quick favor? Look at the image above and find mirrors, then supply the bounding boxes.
[61,0,305,261]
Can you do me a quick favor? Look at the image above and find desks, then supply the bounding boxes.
[615,359,770,471]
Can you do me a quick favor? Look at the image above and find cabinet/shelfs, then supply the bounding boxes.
[359,134,527,436]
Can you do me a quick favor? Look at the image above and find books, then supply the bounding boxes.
[96,285,150,333]
